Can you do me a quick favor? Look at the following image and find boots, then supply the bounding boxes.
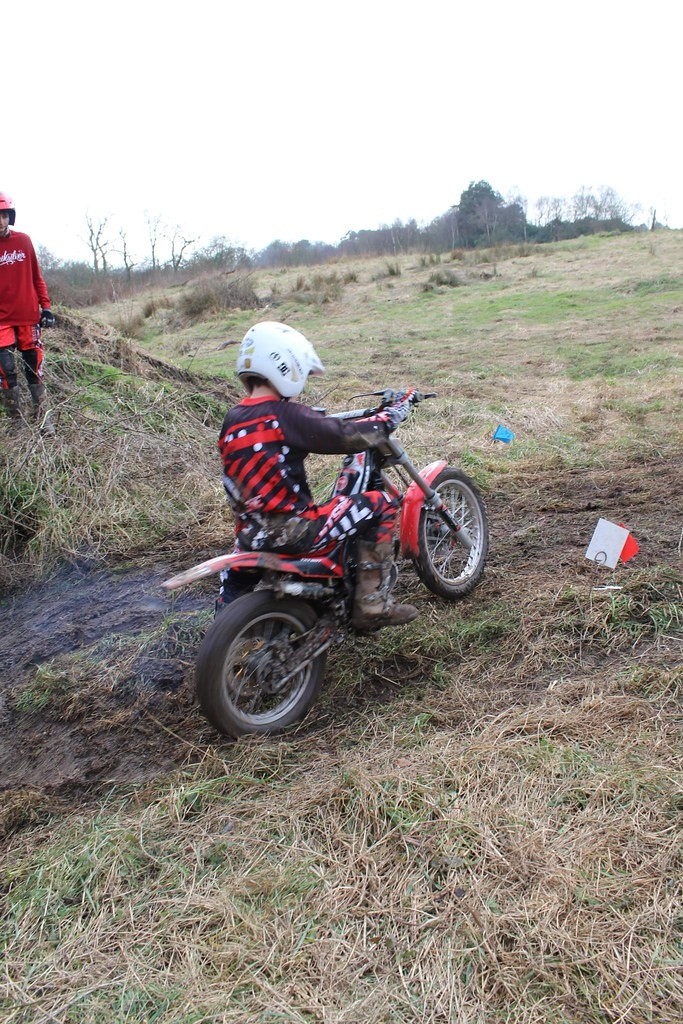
[351,537,420,631]
[3,385,21,437]
[28,384,55,436]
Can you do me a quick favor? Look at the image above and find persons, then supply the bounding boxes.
[0,191,56,440]
[217,320,419,631]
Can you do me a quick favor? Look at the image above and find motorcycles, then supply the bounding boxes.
[159,390,489,745]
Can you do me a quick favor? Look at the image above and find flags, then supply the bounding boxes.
[493,425,515,443]
[617,522,639,564]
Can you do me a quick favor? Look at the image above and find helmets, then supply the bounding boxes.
[237,321,326,398]
[0,192,16,226]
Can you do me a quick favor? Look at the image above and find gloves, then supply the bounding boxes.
[392,390,419,414]
[39,310,54,328]
